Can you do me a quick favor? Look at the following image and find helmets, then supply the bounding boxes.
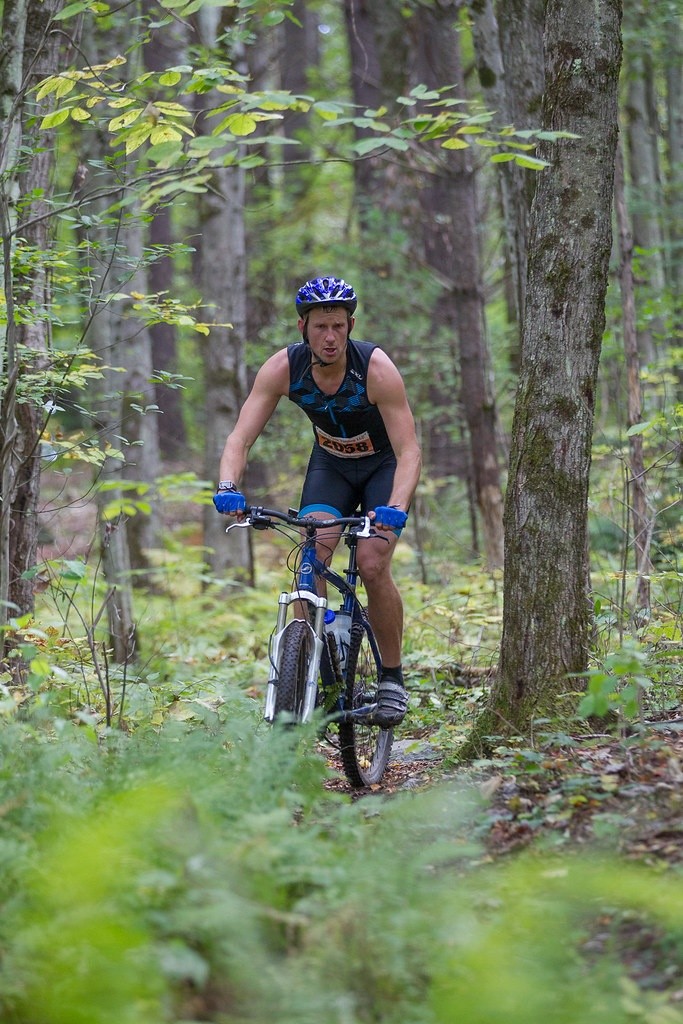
[296,276,357,319]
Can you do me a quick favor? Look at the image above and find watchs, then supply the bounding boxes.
[217,480,237,494]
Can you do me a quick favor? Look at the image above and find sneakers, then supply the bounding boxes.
[373,665,409,728]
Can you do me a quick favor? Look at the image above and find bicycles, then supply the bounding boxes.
[225,504,395,788]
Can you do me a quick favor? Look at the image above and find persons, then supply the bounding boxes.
[213,276,423,727]
